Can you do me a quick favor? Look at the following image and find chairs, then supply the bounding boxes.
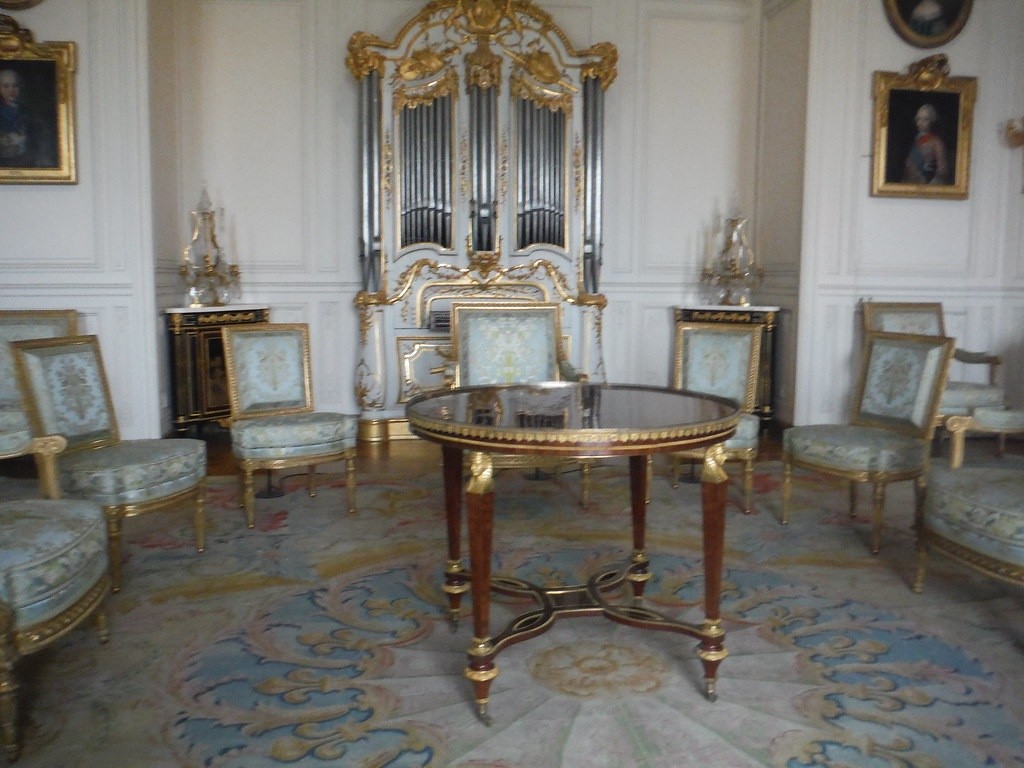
[780,329,956,555]
[451,301,591,510]
[1,309,79,460]
[644,321,762,515]
[8,333,208,594]
[861,300,1007,458]
[220,323,360,529]
[0,435,114,764]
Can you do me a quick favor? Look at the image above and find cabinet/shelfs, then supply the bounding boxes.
[158,303,272,438]
[672,304,782,441]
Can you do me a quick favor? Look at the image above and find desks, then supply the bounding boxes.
[404,380,742,728]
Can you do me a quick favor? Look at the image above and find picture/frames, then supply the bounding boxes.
[870,52,977,200]
[881,0,974,49]
[0,13,79,186]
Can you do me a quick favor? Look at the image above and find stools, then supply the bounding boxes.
[943,405,1024,470]
[911,466,1024,595]
